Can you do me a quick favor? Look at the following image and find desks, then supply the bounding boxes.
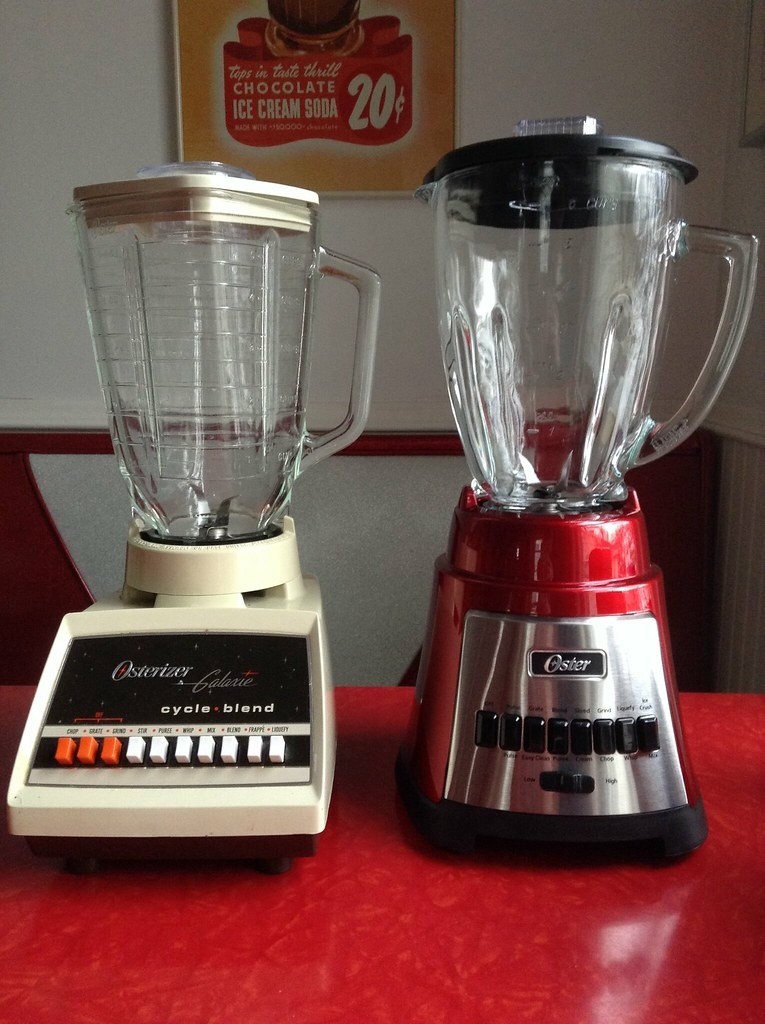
[0,684,765,1024]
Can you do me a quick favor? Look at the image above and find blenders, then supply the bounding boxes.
[407,113,759,863]
[5,160,382,875]
